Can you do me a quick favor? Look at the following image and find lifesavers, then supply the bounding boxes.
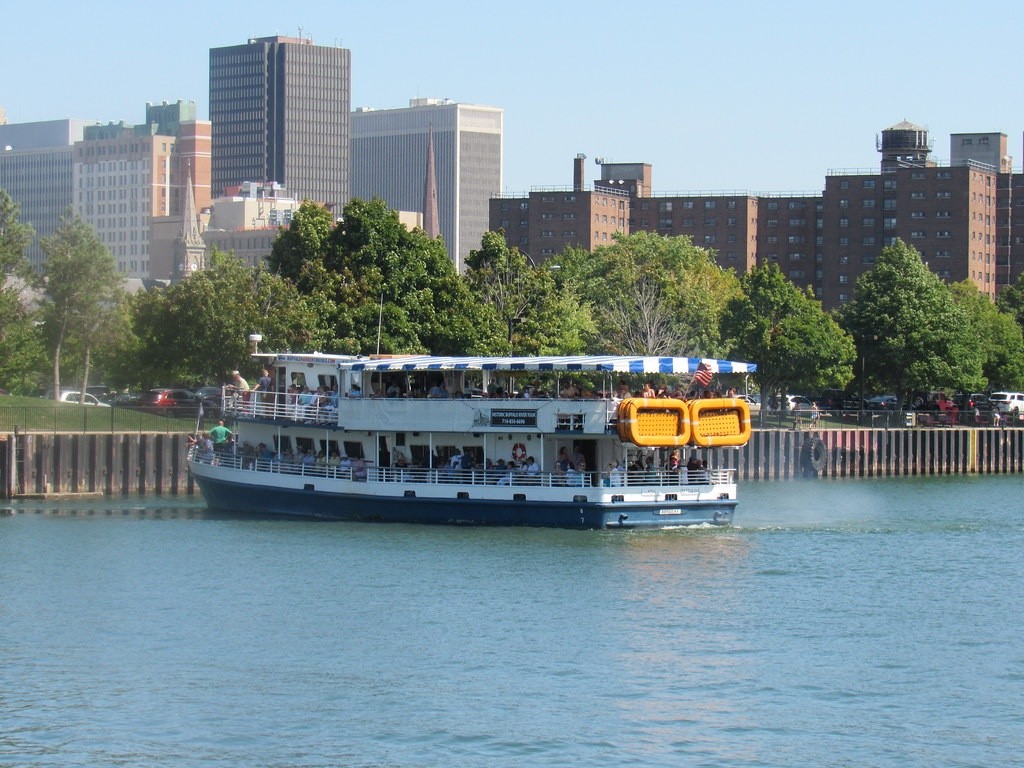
[802,438,828,471]
[512,444,527,460]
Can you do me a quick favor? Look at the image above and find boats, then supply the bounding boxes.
[182,330,759,531]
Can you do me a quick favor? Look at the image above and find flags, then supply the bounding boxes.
[694,363,713,387]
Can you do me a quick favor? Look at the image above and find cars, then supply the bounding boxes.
[722,389,1024,427]
[32,384,234,419]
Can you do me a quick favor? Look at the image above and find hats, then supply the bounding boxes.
[526,456,534,462]
[617,380,626,386]
[497,459,505,462]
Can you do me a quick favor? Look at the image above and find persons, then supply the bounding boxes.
[809,402,820,429]
[348,384,360,397]
[553,445,587,487]
[431,381,449,398]
[392,448,540,485]
[488,376,548,398]
[370,379,401,400]
[1014,406,1019,427]
[603,450,707,487]
[250,369,274,419]
[559,382,574,398]
[992,408,999,426]
[286,385,338,426]
[618,379,735,399]
[237,441,366,480]
[229,371,251,417]
[183,420,234,466]
[795,402,802,430]
